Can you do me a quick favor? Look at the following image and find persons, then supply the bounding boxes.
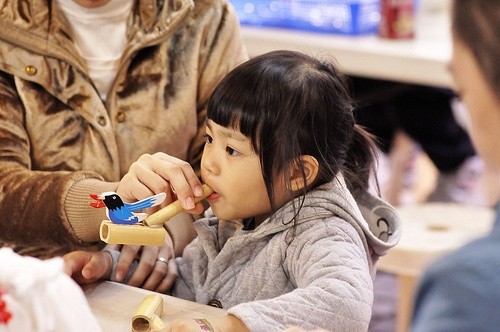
[402,0,500,332]
[0,0,248,297]
[63,50,402,331]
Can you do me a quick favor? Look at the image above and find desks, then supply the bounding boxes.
[238,1,461,91]
[80,278,230,331]
[376,200,497,332]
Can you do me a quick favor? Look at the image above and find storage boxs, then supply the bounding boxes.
[227,1,422,37]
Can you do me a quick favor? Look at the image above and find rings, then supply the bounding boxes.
[156,257,168,264]
